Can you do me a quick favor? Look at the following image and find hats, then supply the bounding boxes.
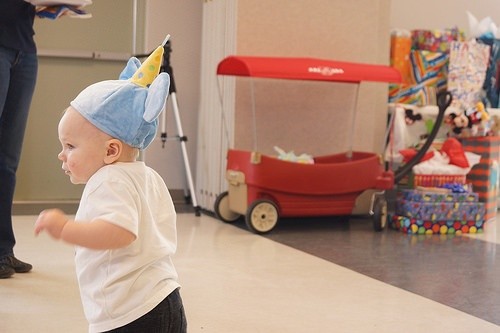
[70,34,171,150]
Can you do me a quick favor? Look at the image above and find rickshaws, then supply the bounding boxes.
[213,54,452,234]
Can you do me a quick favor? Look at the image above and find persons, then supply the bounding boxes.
[33,46,188,333]
[0,0,95,278]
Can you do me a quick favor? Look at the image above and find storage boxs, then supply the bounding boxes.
[387,188,485,235]
[388,30,455,106]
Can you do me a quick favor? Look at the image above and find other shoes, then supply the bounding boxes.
[0,262,16,279]
[0,255,33,273]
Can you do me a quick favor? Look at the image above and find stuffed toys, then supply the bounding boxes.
[443,103,490,134]
